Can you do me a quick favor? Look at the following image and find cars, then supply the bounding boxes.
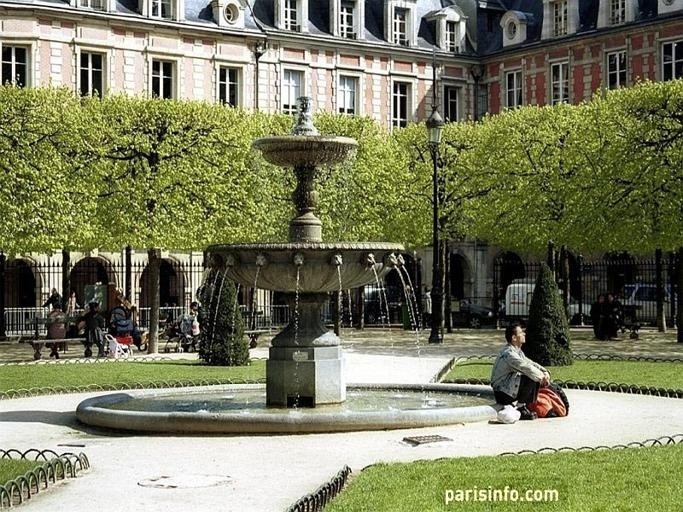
[452,299,493,329]
[330,284,402,323]
[618,284,678,327]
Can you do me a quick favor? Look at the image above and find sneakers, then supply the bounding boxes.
[517,405,536,420]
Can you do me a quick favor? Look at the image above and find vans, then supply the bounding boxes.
[505,278,592,328]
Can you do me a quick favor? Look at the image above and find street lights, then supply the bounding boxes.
[426,108,447,343]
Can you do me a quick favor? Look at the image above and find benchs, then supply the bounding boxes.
[26,317,92,360]
[243,330,270,348]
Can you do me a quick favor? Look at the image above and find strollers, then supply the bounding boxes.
[106,306,134,356]
[165,314,199,353]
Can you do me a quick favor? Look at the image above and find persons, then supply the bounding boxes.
[590,294,607,336]
[606,291,626,338]
[41,288,200,359]
[421,285,432,328]
[489,323,550,419]
